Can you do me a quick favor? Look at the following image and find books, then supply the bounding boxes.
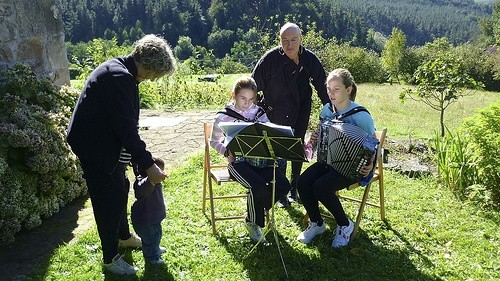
[219,118,306,160]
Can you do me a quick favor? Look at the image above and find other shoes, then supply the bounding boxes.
[278,195,289,208]
[152,258,162,264]
[289,191,302,204]
[159,246,166,253]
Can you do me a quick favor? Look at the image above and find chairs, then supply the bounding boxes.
[202,122,272,234]
[304,128,388,245]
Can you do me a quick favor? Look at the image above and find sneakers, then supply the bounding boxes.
[103,254,139,275]
[119,233,143,248]
[244,218,266,243]
[297,221,326,244]
[332,219,354,247]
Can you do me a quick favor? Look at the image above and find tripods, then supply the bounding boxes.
[230,131,308,279]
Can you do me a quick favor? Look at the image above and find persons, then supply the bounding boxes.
[295,69,378,247]
[209,77,292,242]
[66,35,178,277]
[130,154,169,265]
[251,23,330,207]
